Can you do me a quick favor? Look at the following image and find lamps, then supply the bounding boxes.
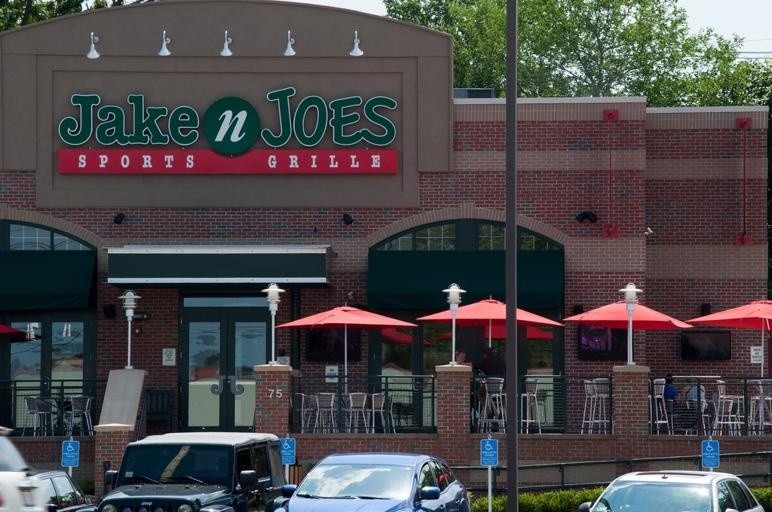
[87,32,100,59]
[159,30,170,56]
[113,212,124,225]
[284,32,295,57]
[340,213,355,225]
[220,31,233,57]
[348,30,363,57]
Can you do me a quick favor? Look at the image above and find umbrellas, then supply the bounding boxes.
[432,324,554,341]
[562,299,694,330]
[683,300,772,377]
[382,327,433,346]
[415,295,565,348]
[0,324,26,337]
[275,301,420,432]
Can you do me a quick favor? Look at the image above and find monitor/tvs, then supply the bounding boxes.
[681,330,731,361]
[577,326,634,362]
[306,329,361,362]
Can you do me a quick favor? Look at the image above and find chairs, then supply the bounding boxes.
[384,394,397,434]
[482,376,505,435]
[748,379,772,434]
[313,393,337,435]
[654,378,670,435]
[522,379,542,434]
[477,378,499,434]
[21,393,97,438]
[590,377,611,434]
[685,385,711,436]
[343,392,368,433]
[296,392,312,434]
[366,393,385,434]
[309,395,318,417]
[712,380,743,435]
[646,379,653,433]
[580,379,599,434]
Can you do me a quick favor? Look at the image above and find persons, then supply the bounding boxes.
[660,372,705,435]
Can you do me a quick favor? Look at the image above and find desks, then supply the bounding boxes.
[672,375,721,433]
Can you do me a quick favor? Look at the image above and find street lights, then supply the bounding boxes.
[441,284,466,367]
[619,282,644,366]
[261,283,287,366]
[118,289,142,370]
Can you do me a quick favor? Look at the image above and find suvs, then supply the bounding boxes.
[96,433,288,512]
[0,426,46,512]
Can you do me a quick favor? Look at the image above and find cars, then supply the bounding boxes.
[33,470,96,512]
[274,452,470,512]
[579,470,765,512]
